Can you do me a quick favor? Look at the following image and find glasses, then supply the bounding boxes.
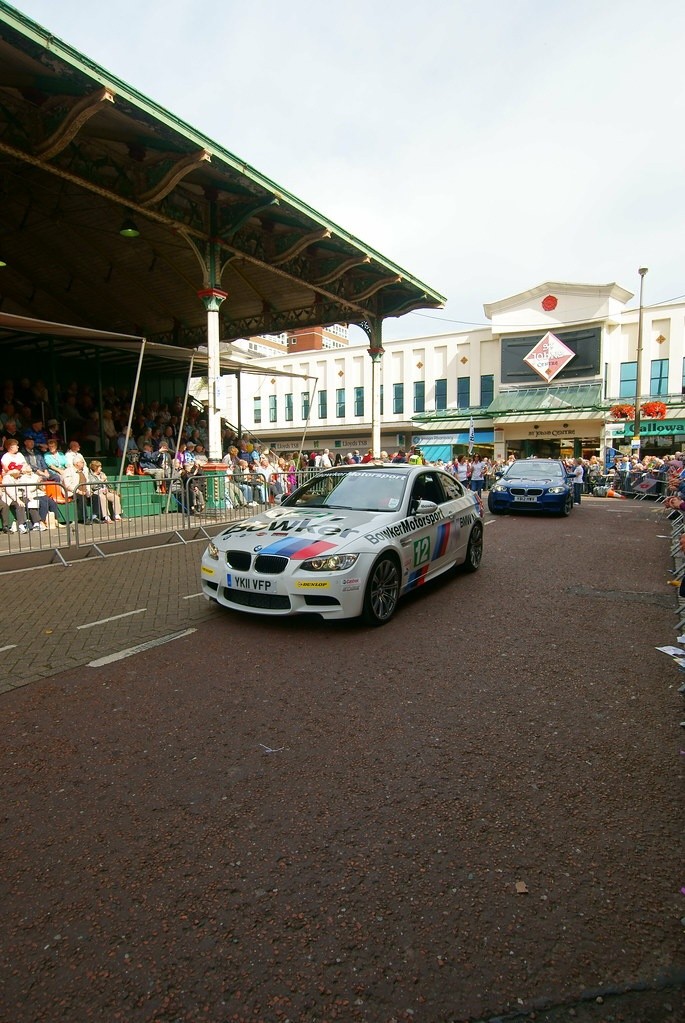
[475,456,479,458]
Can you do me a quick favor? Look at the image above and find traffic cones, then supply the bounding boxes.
[605,488,624,499]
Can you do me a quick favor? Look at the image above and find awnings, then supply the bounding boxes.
[0,309,318,514]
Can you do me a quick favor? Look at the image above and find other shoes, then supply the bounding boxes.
[114,518,126,521]
[253,501,259,505]
[58,524,65,528]
[93,518,104,524]
[574,503,579,505]
[40,524,47,531]
[105,520,113,524]
[32,523,39,531]
[19,524,29,533]
[85,520,91,525]
[249,503,256,507]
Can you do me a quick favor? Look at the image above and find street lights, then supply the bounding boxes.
[630,266,651,457]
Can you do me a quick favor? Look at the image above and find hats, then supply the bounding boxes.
[186,442,196,447]
[143,440,154,446]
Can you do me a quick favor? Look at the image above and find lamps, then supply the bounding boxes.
[120,219,140,237]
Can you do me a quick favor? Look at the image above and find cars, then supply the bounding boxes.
[487,457,578,518]
[200,464,487,628]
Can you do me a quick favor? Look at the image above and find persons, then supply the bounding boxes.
[470,453,487,498]
[428,455,515,490]
[572,460,584,506]
[564,451,685,598]
[269,444,426,496]
[451,455,470,488]
[0,376,291,535]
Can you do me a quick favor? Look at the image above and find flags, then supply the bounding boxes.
[467,420,475,455]
